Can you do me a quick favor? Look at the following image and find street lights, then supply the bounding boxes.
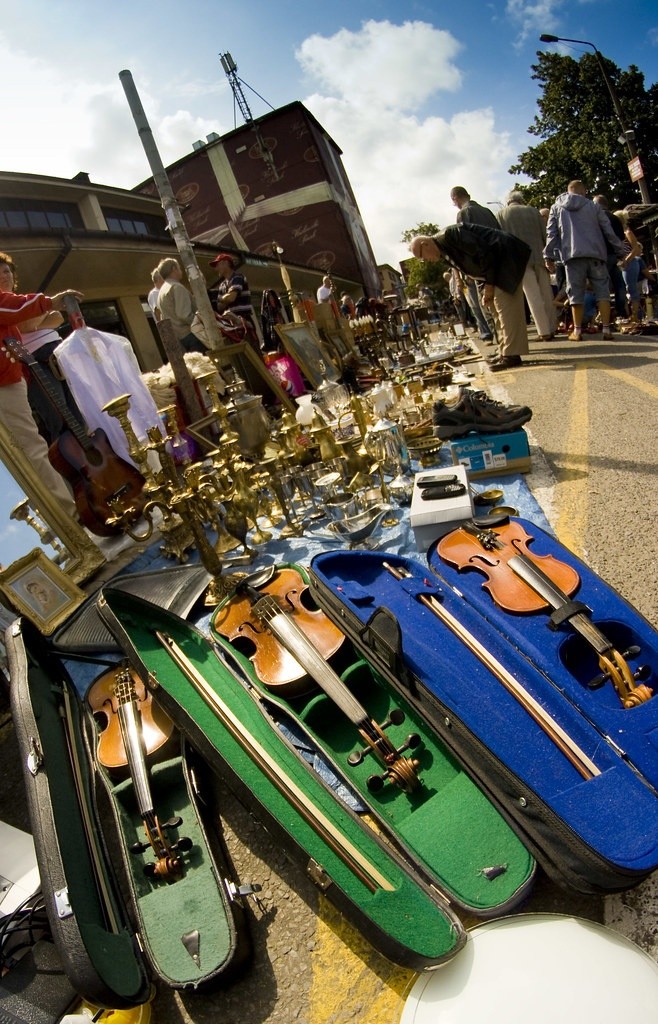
[538,33,658,267]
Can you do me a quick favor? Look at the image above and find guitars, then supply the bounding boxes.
[3,337,153,540]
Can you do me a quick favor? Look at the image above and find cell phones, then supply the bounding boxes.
[421,484,465,500]
[417,475,458,488]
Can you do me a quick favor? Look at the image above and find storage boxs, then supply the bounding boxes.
[451,428,531,481]
[410,465,475,552]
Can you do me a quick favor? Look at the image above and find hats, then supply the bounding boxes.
[209,255,233,267]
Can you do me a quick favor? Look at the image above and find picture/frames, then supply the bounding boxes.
[273,320,342,389]
[185,342,297,451]
[0,546,89,636]
[325,330,354,365]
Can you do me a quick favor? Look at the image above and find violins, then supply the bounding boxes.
[82,656,191,883]
[435,508,653,714]
[208,565,434,804]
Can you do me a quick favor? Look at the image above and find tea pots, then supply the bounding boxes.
[368,384,392,417]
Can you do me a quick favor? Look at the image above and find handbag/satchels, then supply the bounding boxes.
[263,347,304,396]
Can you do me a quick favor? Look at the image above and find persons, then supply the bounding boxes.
[147,253,282,419]
[316,180,658,372]
[0,254,86,524]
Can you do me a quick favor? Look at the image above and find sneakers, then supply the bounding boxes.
[433,388,531,441]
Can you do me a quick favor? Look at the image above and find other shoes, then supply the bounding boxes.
[535,332,555,341]
[486,357,500,364]
[568,332,581,340]
[489,356,523,371]
[603,332,613,339]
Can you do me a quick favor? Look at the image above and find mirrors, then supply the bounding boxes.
[0,423,107,618]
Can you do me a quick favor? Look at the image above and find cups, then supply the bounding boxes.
[328,416,356,442]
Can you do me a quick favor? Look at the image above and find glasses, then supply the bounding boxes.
[419,244,424,263]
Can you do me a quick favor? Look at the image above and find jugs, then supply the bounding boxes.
[364,416,410,476]
